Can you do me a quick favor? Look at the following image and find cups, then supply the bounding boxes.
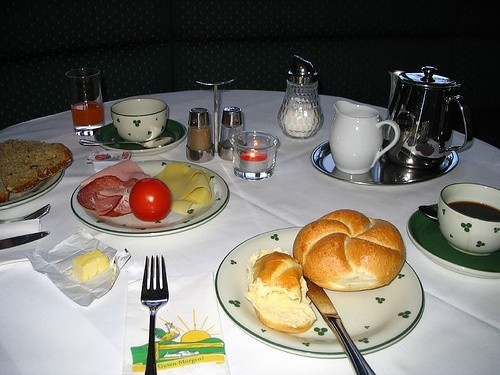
[66,67,107,136]
[227,131,279,180]
[329,98,400,174]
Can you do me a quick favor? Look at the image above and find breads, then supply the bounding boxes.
[0,139,74,203]
[293,209,407,292]
[244,247,317,333]
[72,249,109,282]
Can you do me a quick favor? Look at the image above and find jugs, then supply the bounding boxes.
[386,66,474,174]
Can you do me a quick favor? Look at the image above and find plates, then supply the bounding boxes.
[312,137,458,185]
[407,204,500,280]
[215,227,425,357]
[0,170,65,210]
[71,160,230,237]
[97,119,187,156]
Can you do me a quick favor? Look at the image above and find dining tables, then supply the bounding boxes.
[0,90,500,375]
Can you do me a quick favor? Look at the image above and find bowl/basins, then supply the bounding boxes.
[111,98,170,141]
[437,181,500,256]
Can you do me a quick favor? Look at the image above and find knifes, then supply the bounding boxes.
[304,276,376,375]
[0,231,49,251]
[0,204,51,225]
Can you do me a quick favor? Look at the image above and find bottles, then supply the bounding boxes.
[187,108,215,163]
[219,106,246,160]
[277,55,323,139]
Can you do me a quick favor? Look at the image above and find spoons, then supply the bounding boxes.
[78,136,172,148]
[419,206,439,221]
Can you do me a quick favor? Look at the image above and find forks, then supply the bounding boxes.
[140,254,169,375]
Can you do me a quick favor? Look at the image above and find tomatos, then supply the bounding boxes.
[129,178,173,222]
